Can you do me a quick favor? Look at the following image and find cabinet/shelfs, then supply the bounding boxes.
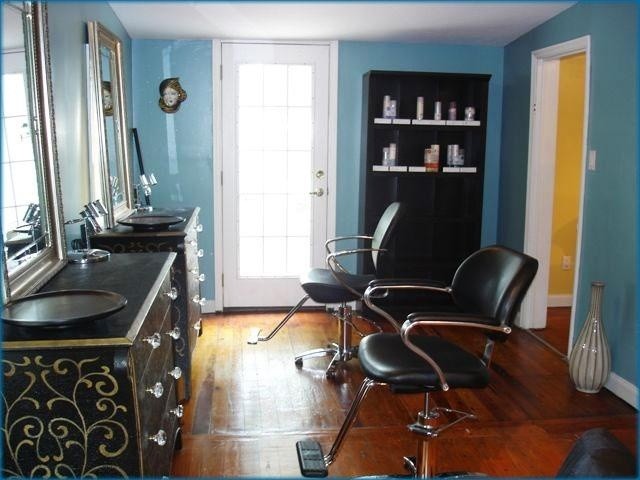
[356,70,492,319]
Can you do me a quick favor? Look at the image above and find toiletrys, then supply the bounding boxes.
[381,95,475,168]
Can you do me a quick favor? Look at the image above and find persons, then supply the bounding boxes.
[161,80,187,108]
[100,82,115,116]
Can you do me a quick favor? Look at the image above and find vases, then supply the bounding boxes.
[568,282,612,394]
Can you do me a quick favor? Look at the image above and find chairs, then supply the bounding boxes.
[556,427,636,480]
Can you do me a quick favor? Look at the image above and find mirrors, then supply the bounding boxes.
[87,19,132,230]
[0,1,69,307]
